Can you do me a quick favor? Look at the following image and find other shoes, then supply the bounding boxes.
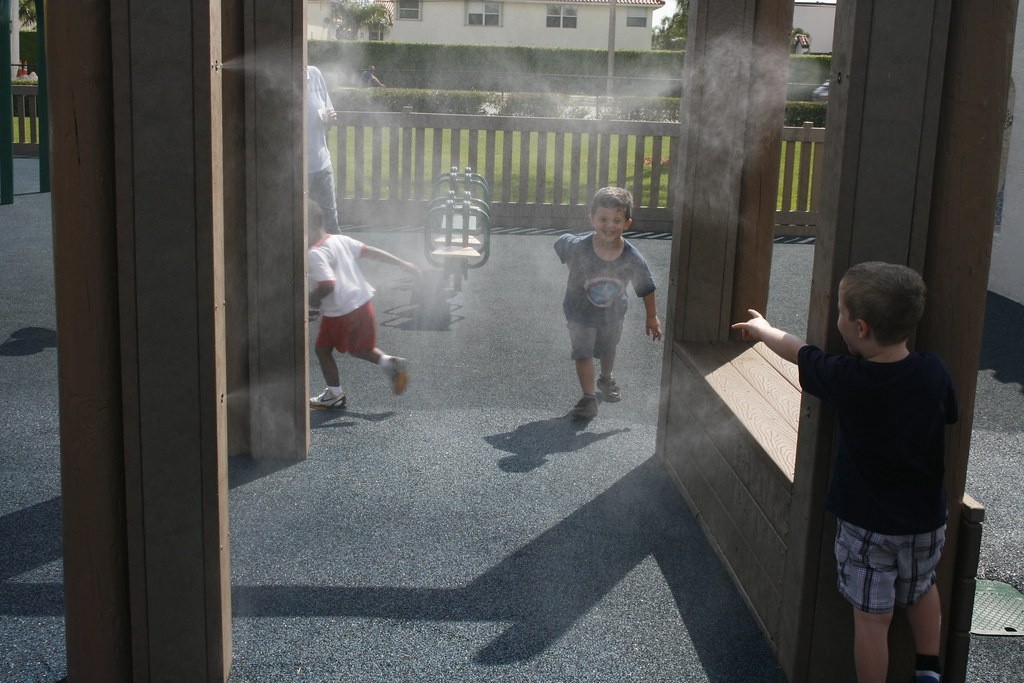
[915,654,941,683]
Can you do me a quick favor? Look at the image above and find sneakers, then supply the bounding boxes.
[572,394,598,417]
[596,374,622,402]
[389,357,409,395]
[310,389,347,408]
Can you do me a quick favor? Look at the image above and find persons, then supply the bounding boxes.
[731,262,960,683]
[307,65,341,320]
[306,198,421,406]
[357,65,384,89]
[555,186,667,417]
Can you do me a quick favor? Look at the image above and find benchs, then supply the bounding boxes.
[655,339,987,683]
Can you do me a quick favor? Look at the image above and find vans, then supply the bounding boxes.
[810,79,829,102]
